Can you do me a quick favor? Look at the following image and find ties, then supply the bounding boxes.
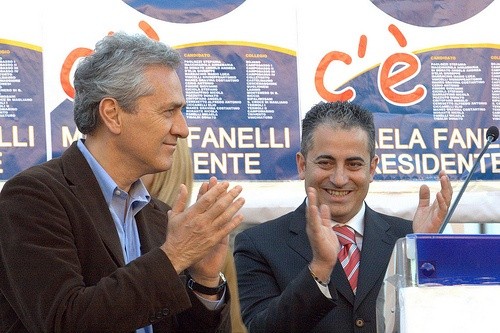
[332,224,361,297]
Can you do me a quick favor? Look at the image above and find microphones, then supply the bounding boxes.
[438,126,499,234]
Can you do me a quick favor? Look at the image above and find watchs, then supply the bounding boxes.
[187,271,226,294]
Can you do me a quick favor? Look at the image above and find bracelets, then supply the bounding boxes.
[307,266,330,287]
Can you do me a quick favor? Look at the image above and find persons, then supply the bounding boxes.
[236,102,451,332]
[0,35,243,333]
